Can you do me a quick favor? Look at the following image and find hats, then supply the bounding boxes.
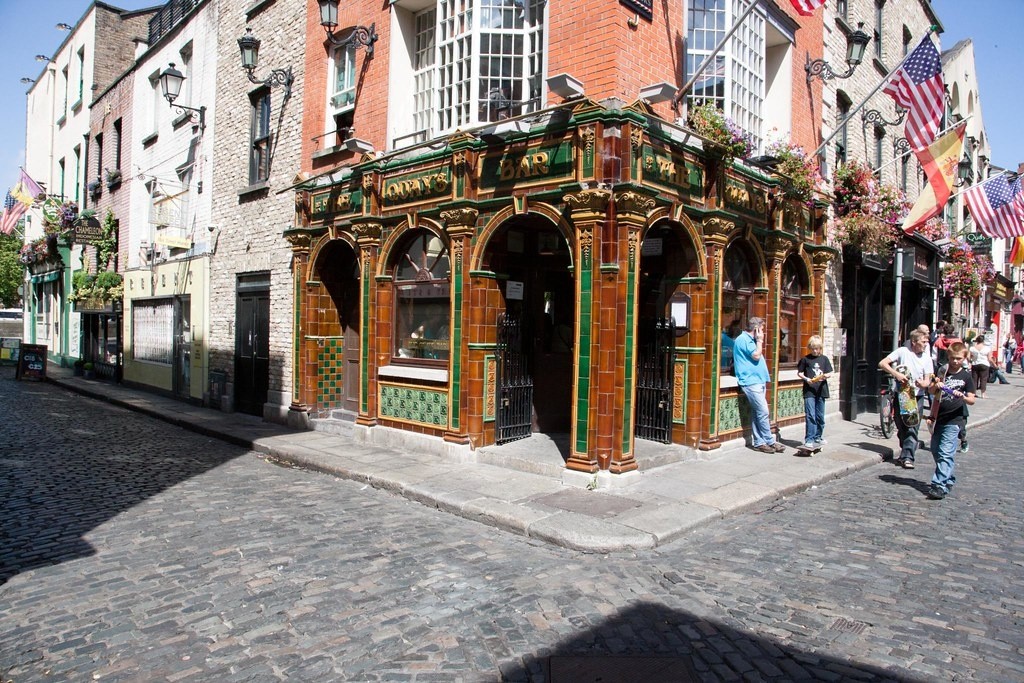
[748,317,763,329]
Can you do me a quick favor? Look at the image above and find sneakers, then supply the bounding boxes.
[898,458,902,461]
[959,440,969,453]
[754,444,775,452]
[815,438,827,444]
[805,442,813,447]
[902,460,915,468]
[770,443,785,452]
[946,484,952,491]
[928,484,945,498]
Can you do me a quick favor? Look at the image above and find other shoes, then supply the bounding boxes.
[1000,381,1010,384]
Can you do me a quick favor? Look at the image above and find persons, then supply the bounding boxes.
[797,335,835,448]
[969,335,998,399]
[902,313,1024,453]
[720,329,736,373]
[878,328,935,470]
[924,343,975,500]
[732,317,786,454]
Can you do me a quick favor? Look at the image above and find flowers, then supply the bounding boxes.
[765,140,821,208]
[827,160,995,299]
[692,101,752,160]
[20,171,119,268]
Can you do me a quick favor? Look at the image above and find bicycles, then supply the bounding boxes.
[878,369,897,439]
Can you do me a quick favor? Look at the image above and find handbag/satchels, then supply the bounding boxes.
[987,367,996,383]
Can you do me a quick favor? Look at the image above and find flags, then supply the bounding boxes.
[1008,174,1024,221]
[1009,237,1024,266]
[902,121,968,236]
[0,165,44,235]
[965,172,1024,238]
[789,0,828,16]
[881,24,943,154]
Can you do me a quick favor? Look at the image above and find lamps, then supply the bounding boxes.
[55,23,73,31]
[547,73,583,98]
[750,156,779,166]
[34,55,55,64]
[236,27,293,98]
[19,78,34,83]
[805,23,872,83]
[638,82,680,105]
[344,138,374,154]
[159,63,206,130]
[861,101,908,131]
[316,0,378,61]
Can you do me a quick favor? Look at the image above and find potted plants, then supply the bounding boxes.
[84,362,93,378]
